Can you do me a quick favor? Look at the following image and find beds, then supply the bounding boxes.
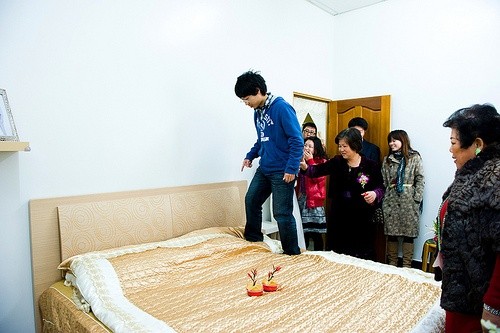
[29,180,446,333]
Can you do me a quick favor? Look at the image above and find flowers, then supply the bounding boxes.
[248,269,259,286]
[267,266,282,281]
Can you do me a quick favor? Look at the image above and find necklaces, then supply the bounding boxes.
[438,196,448,251]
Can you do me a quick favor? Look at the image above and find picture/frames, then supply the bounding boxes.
[0,89,19,141]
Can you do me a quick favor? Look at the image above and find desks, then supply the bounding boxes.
[422,239,440,273]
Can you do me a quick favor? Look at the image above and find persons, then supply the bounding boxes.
[234,71,304,256]
[295,136,329,251]
[432,104,500,333]
[381,130,425,268]
[302,122,317,138]
[300,128,386,261]
[348,117,380,168]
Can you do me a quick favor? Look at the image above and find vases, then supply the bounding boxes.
[263,281,277,292]
[246,287,263,297]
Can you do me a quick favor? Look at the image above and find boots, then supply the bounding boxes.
[402,243,413,268]
[386,242,398,266]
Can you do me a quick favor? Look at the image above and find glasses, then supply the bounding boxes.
[304,144,314,149]
[303,129,315,134]
[240,94,253,104]
